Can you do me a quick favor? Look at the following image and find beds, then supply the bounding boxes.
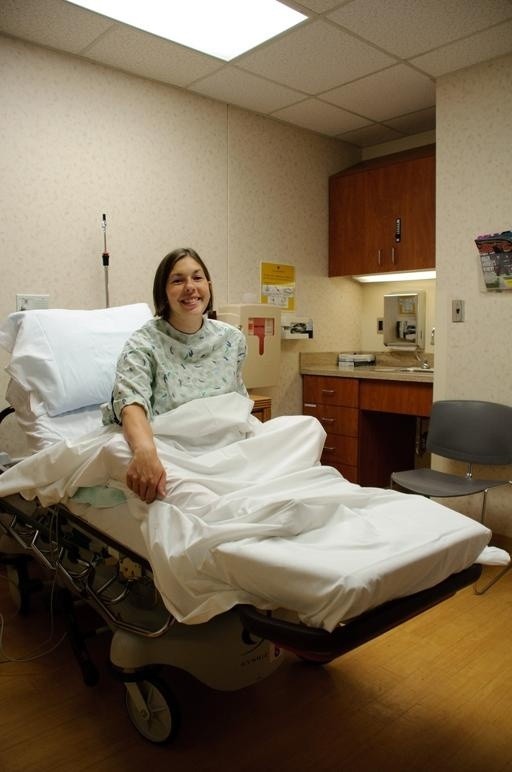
[0,375,494,660]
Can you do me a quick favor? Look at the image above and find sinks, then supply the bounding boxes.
[399,368,434,374]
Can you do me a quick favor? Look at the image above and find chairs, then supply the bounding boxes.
[388,400,512,596]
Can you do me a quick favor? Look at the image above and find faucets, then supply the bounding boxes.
[414,353,430,369]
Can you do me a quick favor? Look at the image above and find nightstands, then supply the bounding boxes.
[248,394,271,425]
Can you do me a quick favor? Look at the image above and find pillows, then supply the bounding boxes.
[10,302,154,418]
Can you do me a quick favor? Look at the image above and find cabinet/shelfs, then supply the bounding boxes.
[300,370,432,489]
[327,143,435,276]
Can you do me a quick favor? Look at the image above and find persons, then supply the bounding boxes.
[100,248,249,504]
